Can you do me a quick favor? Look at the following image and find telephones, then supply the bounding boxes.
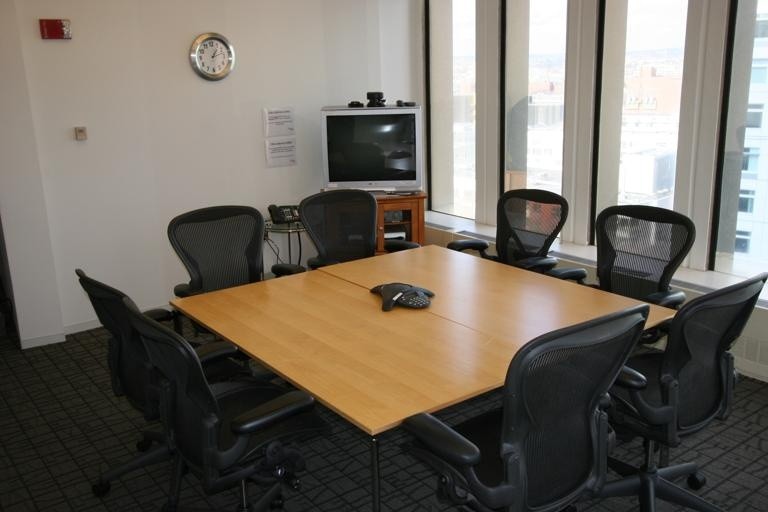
[267,205,301,224]
[370,283,435,311]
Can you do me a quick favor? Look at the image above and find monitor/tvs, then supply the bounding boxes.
[320,105,423,192]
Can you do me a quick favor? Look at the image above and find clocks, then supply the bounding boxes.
[189,33,235,81]
